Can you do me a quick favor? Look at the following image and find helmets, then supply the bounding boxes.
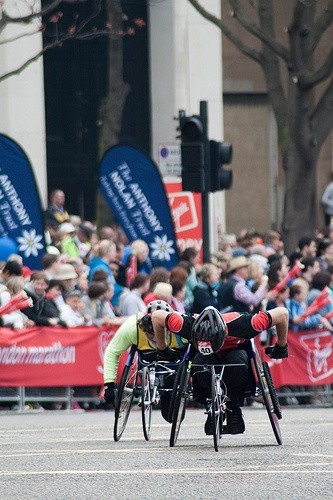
[144,300,172,335]
[192,306,226,355]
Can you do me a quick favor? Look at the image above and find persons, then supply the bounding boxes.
[43,189,70,230]
[320,180,333,227]
[150,305,289,434]
[0,213,171,413]
[165,226,333,408]
[102,300,190,423]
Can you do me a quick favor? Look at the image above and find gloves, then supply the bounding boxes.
[269,344,288,359]
[156,347,178,361]
[103,386,114,403]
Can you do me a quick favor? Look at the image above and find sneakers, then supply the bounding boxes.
[204,413,225,435]
[227,412,244,434]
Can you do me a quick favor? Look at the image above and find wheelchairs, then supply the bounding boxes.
[113,344,282,451]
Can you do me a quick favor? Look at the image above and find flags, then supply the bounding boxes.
[97,143,181,271]
[0,132,48,271]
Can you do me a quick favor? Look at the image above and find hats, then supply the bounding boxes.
[58,222,75,234]
[227,255,249,273]
[53,264,76,279]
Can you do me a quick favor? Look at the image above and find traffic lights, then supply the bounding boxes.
[180,109,206,195]
[210,139,233,192]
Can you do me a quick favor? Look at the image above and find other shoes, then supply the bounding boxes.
[159,396,171,422]
[11,396,99,412]
[174,401,185,422]
[246,387,333,409]
